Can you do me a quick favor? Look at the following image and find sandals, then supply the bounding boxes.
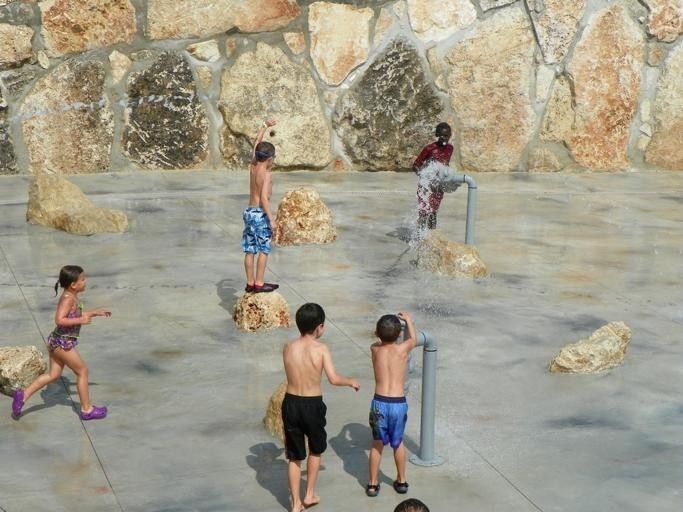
[394,480,408,493]
[365,484,380,496]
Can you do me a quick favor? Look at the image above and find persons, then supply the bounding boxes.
[239,117,279,294]
[412,122,454,231]
[12,265,112,421]
[393,498,430,512]
[365,310,417,497]
[281,302,359,511]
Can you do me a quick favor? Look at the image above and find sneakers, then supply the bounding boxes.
[255,282,279,292]
[245,283,254,292]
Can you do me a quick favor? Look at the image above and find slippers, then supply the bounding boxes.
[12,389,23,416]
[81,405,107,419]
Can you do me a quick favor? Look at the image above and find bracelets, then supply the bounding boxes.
[260,122,267,128]
